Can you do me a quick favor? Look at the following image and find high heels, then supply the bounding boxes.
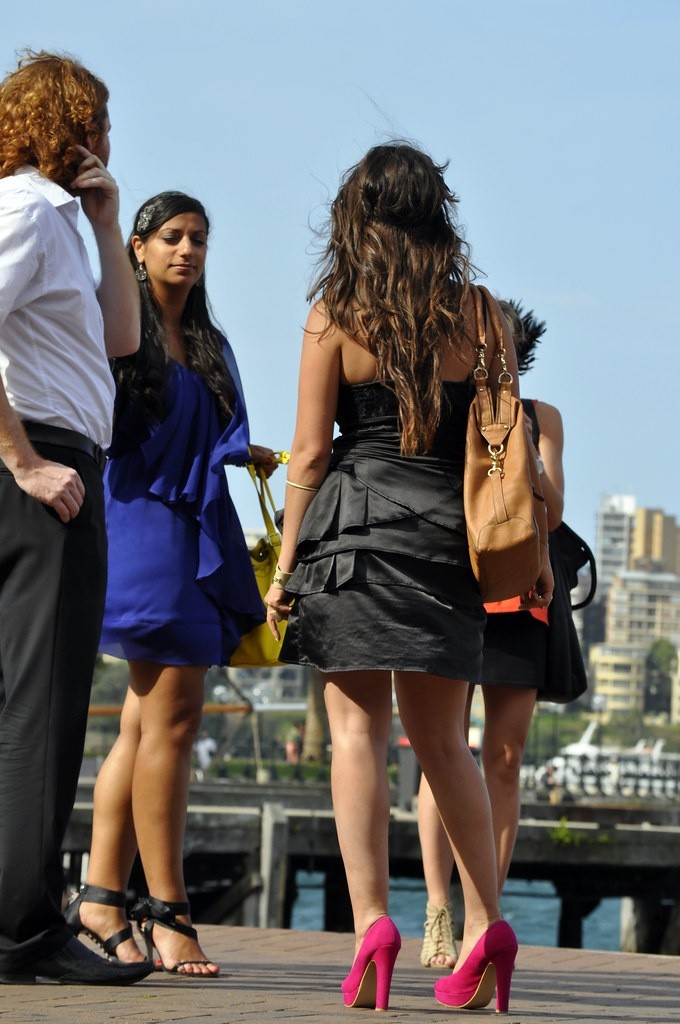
[129,895,219,978]
[434,920,518,1014]
[62,883,147,963]
[341,916,402,1012]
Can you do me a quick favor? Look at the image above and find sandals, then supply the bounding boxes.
[420,900,459,968]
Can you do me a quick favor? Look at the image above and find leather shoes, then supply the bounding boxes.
[0,934,154,986]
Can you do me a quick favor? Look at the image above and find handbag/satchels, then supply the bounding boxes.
[227,446,294,667]
[463,282,542,603]
[519,395,597,703]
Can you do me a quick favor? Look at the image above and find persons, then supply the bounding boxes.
[257,141,519,1012]
[421,288,564,960]
[0,44,153,990]
[63,188,277,975]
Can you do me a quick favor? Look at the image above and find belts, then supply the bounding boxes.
[22,420,109,473]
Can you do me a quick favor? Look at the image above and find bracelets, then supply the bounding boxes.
[273,563,294,589]
[286,481,319,495]
